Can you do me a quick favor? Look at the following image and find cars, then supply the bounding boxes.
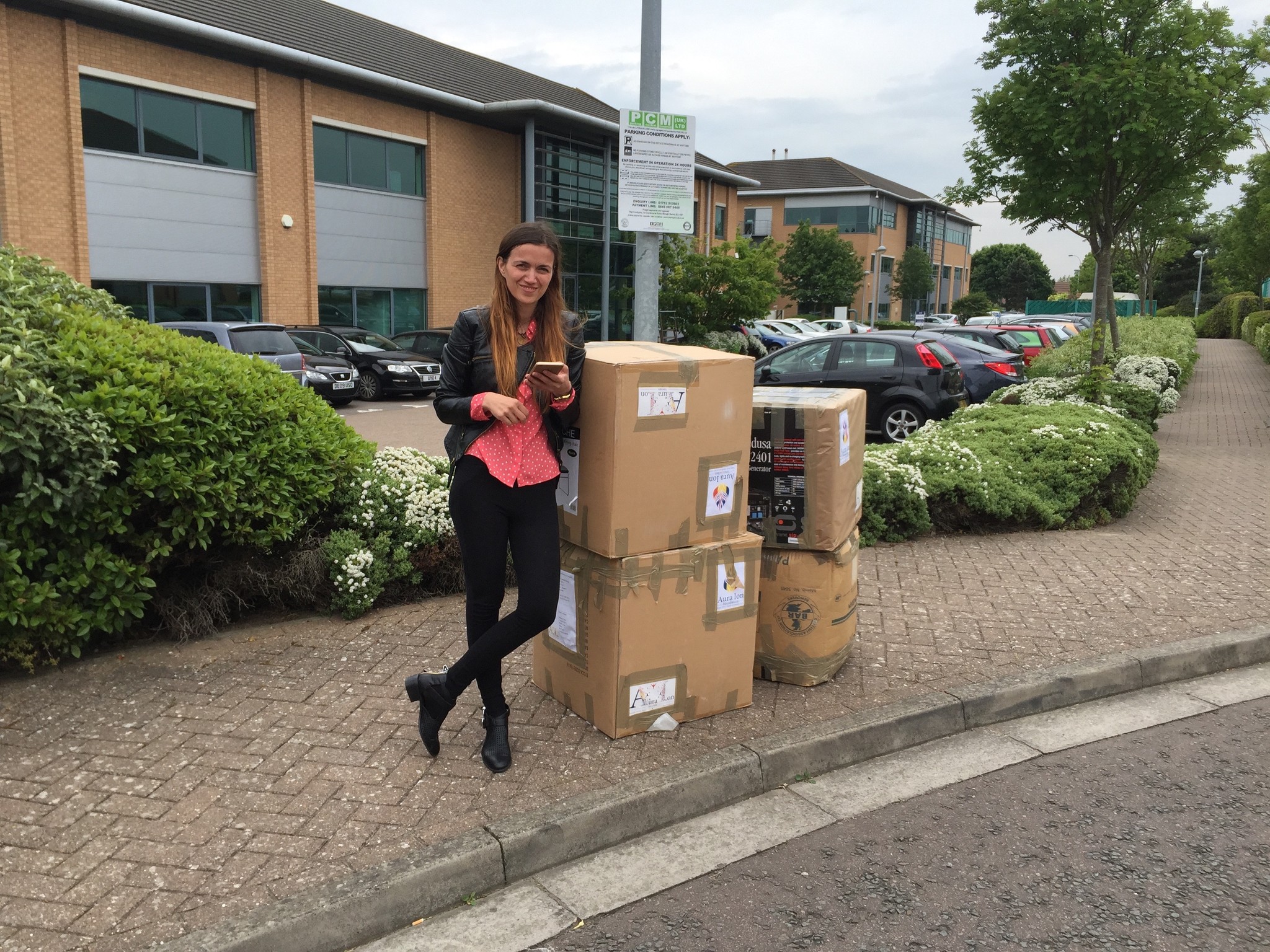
[918,329,1025,361]
[285,334,362,404]
[927,314,961,325]
[907,318,961,330]
[320,303,419,330]
[376,329,452,361]
[989,310,1092,342]
[668,314,827,360]
[855,322,879,332]
[953,324,1064,367]
[753,332,970,443]
[811,319,859,334]
[965,316,1003,326]
[801,329,1028,406]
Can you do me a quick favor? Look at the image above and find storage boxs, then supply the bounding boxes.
[533,339,866,739]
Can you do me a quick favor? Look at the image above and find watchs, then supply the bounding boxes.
[554,387,573,401]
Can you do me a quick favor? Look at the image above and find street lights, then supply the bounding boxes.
[1083,238,1100,338]
[1068,254,1082,294]
[1193,249,1209,328]
[860,271,869,324]
[870,246,887,331]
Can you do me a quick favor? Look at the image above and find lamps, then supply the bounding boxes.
[876,191,879,198]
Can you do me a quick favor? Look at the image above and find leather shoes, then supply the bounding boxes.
[405,672,457,757]
[480,703,511,773]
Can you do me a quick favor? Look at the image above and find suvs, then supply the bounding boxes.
[285,323,442,401]
[148,320,309,390]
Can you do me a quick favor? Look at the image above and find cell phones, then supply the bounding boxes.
[527,362,564,384]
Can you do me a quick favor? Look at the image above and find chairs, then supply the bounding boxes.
[839,345,854,370]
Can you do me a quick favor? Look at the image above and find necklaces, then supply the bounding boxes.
[518,330,526,339]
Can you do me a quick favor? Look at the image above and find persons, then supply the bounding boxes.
[404,220,586,776]
